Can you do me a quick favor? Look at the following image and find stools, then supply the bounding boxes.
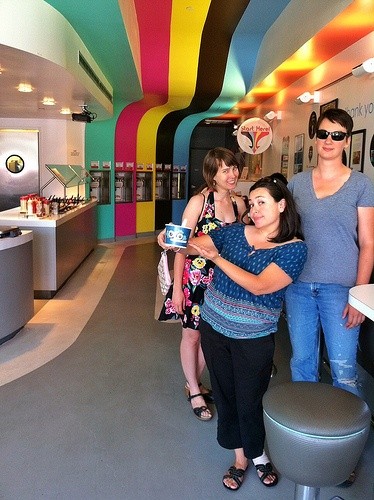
[262,381,371,500]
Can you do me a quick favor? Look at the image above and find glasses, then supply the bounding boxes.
[255,177,281,185]
[315,130,348,141]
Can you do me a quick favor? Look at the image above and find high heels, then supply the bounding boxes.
[187,391,211,420]
[185,383,215,403]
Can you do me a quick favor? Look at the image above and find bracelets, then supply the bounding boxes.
[173,248,180,253]
[211,254,221,261]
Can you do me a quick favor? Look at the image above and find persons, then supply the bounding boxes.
[281,111,374,489]
[159,149,249,421]
[157,174,307,491]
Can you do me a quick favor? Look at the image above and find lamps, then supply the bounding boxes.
[293,91,321,105]
[264,110,282,123]
[351,57,374,79]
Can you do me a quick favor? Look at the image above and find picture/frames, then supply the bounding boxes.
[281,155,288,179]
[349,129,366,173]
[319,98,338,116]
[293,133,305,174]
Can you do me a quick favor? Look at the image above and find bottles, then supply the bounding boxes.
[20,193,59,217]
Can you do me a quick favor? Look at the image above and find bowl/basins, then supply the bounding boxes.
[165,224,192,249]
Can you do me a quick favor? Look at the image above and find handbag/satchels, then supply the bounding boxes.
[157,249,173,296]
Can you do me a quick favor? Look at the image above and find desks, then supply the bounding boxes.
[0,230,33,345]
[0,198,98,300]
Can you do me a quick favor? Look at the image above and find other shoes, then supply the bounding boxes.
[336,471,355,487]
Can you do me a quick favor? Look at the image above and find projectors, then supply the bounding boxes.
[72,113,91,123]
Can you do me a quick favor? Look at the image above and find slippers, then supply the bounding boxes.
[223,466,248,491]
[251,460,278,487]
